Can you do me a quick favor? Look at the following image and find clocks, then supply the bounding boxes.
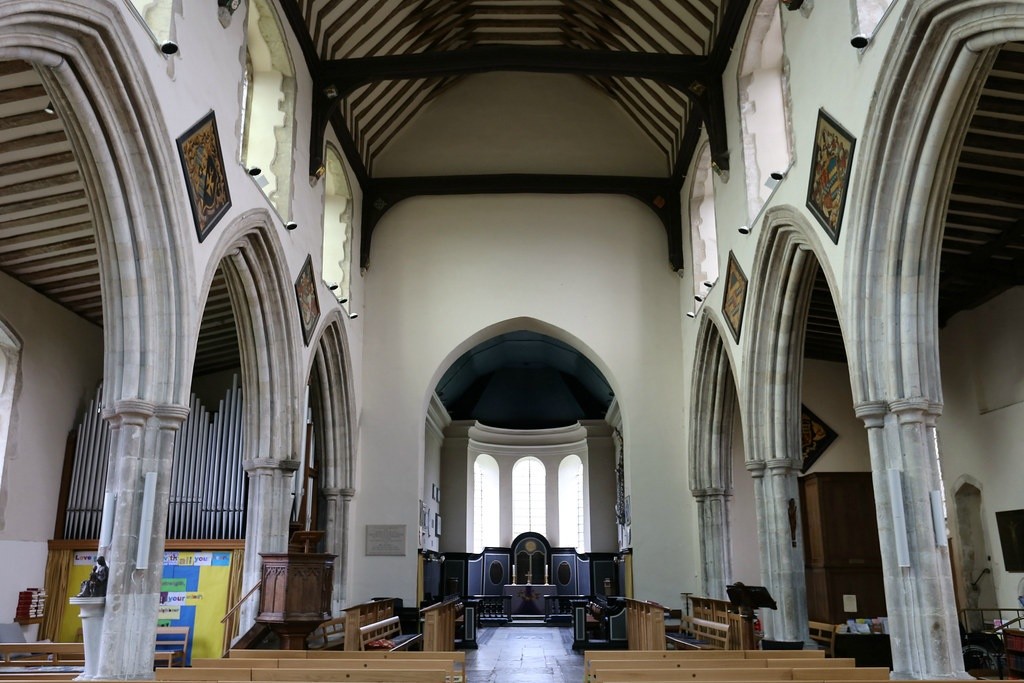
[218,0,241,15]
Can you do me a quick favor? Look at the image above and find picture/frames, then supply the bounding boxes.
[805,107,857,245]
[174,109,233,243]
[294,254,322,345]
[720,250,749,345]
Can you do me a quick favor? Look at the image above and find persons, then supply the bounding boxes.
[76,556,106,597]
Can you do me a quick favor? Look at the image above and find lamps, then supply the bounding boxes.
[329,281,358,320]
[246,166,261,176]
[850,34,872,47]
[686,281,715,318]
[283,221,297,230]
[737,226,752,234]
[770,172,786,180]
[160,40,178,54]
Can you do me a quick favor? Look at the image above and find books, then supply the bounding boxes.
[993,619,1010,633]
[26,588,48,618]
[17,591,38,618]
[872,617,888,634]
[847,619,873,635]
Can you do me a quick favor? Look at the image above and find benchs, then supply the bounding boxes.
[358,615,423,651]
[584,648,891,683]
[664,617,731,650]
[0,642,466,683]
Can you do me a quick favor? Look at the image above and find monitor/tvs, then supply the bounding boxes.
[996,509,1024,572]
